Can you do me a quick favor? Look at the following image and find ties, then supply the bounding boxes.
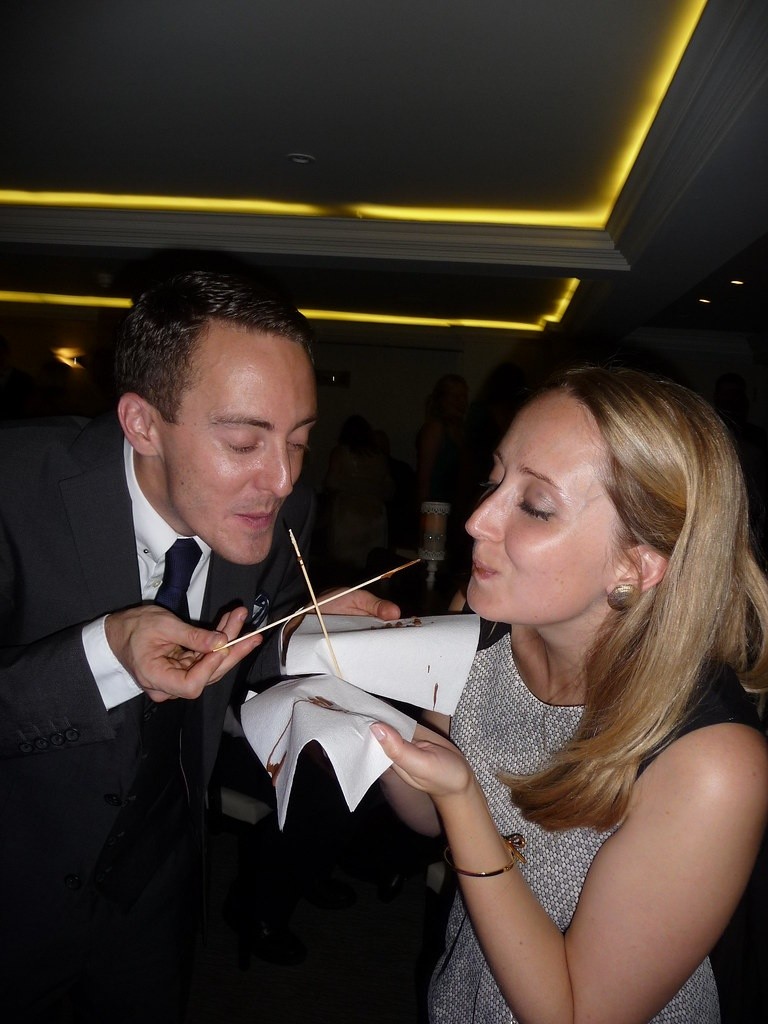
[154,540,197,620]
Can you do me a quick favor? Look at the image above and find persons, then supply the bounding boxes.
[0,263,434,1023]
[288,370,768,1024]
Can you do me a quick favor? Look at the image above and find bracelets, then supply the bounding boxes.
[443,833,527,877]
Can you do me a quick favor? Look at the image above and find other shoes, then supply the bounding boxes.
[223,904,308,966]
[303,877,353,909]
